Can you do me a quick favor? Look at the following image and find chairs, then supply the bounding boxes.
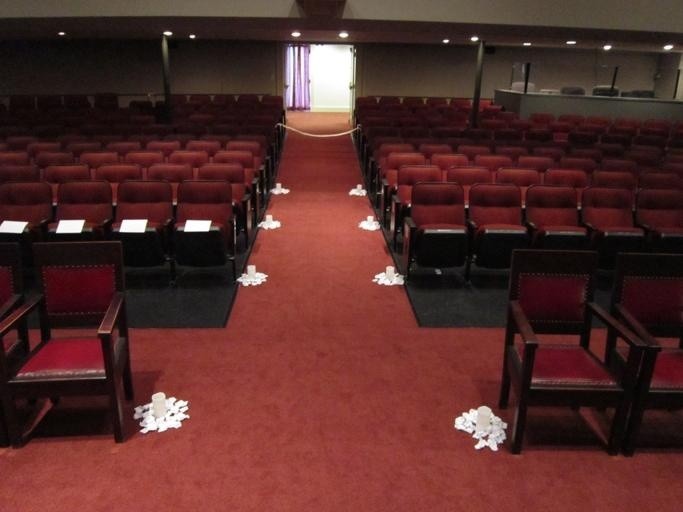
[498,249,648,458]
[355,97,680,289]
[602,254,681,457]
[1,92,286,282]
[1,243,37,411]
[2,239,133,444]
